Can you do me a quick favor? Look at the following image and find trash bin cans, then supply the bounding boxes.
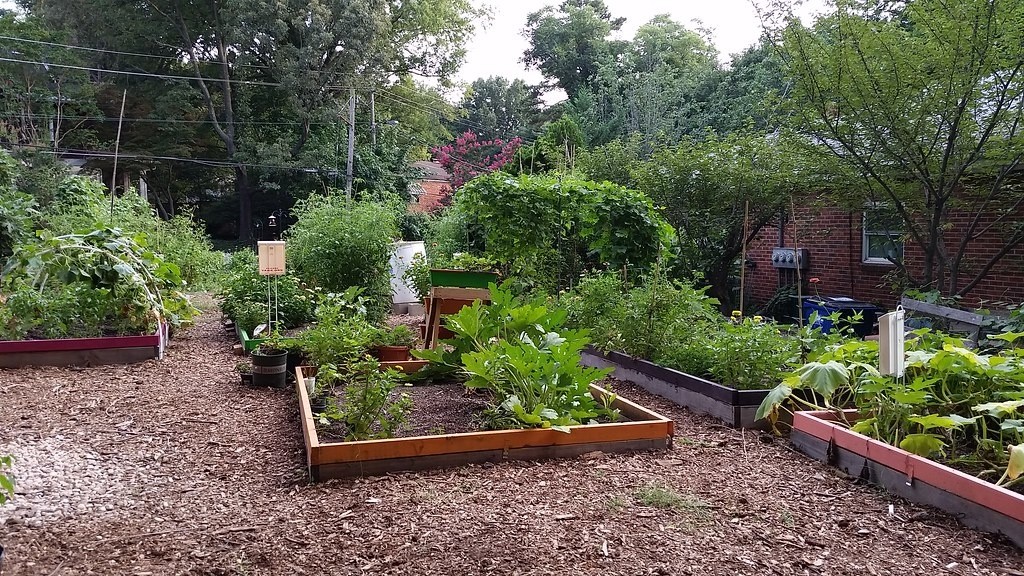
[803,295,876,340]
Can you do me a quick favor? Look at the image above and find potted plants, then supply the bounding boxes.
[240,364,253,385]
[376,324,412,361]
[250,331,289,389]
[429,252,497,289]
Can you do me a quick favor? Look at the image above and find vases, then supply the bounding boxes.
[424,296,492,315]
[420,323,456,341]
